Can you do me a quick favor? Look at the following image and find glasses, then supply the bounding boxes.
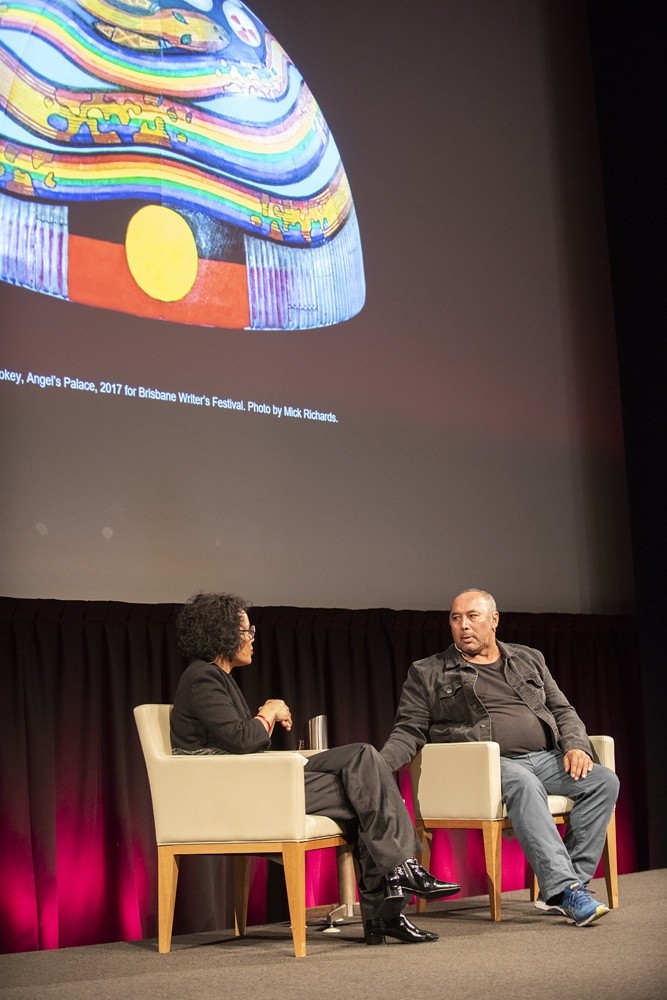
[239,625,255,636]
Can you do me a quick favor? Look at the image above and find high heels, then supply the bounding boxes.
[362,913,439,945]
[385,857,461,901]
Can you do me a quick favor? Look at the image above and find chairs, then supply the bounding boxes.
[410,733,621,922]
[132,703,352,958]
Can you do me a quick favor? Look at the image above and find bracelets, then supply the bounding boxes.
[256,715,271,732]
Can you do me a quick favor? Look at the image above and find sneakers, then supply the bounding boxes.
[535,890,567,915]
[561,881,609,927]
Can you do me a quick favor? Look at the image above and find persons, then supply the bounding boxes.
[379,588,620,927]
[169,588,462,945]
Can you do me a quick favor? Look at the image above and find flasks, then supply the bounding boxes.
[308,714,328,749]
